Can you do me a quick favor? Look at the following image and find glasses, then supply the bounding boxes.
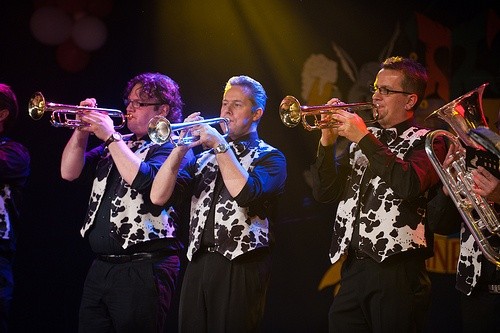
[369,85,411,95]
[123,98,164,108]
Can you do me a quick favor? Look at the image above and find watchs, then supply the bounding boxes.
[214,143,229,154]
[105,132,122,148]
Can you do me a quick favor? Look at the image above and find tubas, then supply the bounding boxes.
[423,81,500,268]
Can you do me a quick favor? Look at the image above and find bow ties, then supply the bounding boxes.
[226,140,250,156]
[124,139,147,149]
[367,127,397,146]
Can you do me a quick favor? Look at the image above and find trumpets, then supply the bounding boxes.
[278,95,381,132]
[146,115,232,148]
[27,91,133,130]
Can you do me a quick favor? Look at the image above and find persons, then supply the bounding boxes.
[426,146,500,333]
[0,82,31,333]
[61,75,194,333]
[150,76,287,333]
[313,56,445,333]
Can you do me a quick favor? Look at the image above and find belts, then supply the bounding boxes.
[347,248,369,261]
[97,252,160,264]
[478,283,500,294]
[200,246,214,253]
